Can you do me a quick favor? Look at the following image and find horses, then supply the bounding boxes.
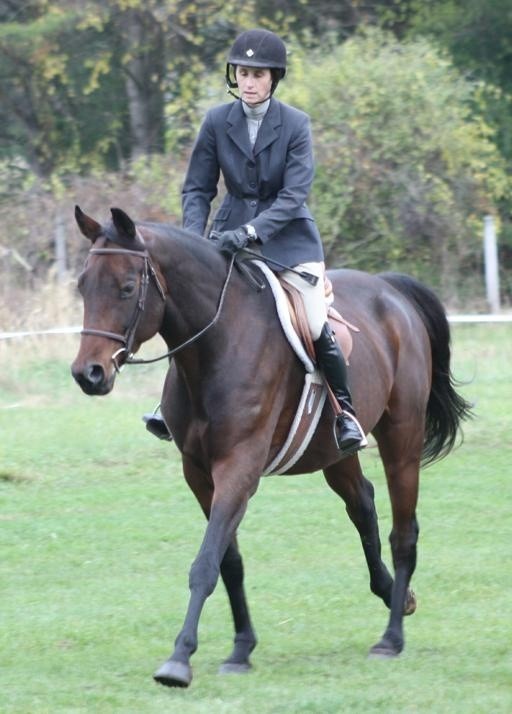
[70,205,481,689]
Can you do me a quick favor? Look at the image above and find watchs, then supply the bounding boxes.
[245,224,258,241]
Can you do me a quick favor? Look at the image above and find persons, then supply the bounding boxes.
[145,30,363,450]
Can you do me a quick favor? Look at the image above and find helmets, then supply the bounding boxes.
[227,28,286,78]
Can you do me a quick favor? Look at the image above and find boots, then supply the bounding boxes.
[311,321,362,450]
[144,414,171,442]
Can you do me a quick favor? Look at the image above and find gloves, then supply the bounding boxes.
[221,224,255,253]
